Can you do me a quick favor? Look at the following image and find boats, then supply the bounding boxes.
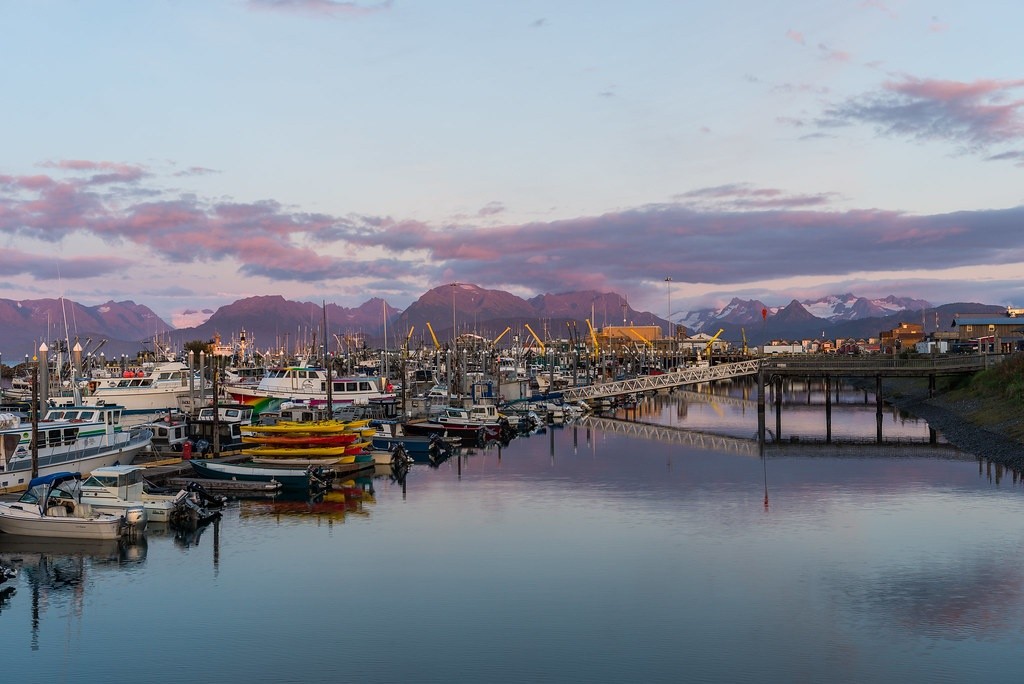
[0,533,148,563]
[0,273,710,540]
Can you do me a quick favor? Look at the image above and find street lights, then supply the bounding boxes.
[450,282,459,343]
[664,277,672,351]
